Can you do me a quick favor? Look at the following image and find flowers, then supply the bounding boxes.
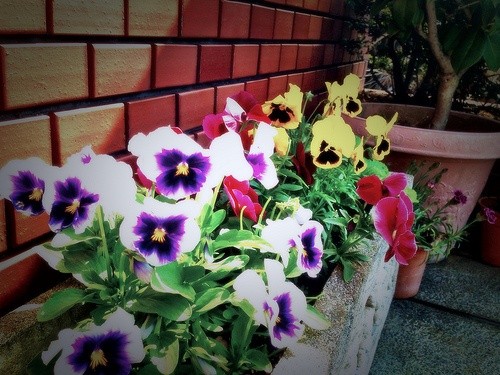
[407,157,498,251]
[0,71,415,375]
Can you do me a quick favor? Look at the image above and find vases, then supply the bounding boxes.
[395,247,430,299]
[477,195,500,267]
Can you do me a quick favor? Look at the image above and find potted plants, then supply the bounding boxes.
[339,0,500,265]
